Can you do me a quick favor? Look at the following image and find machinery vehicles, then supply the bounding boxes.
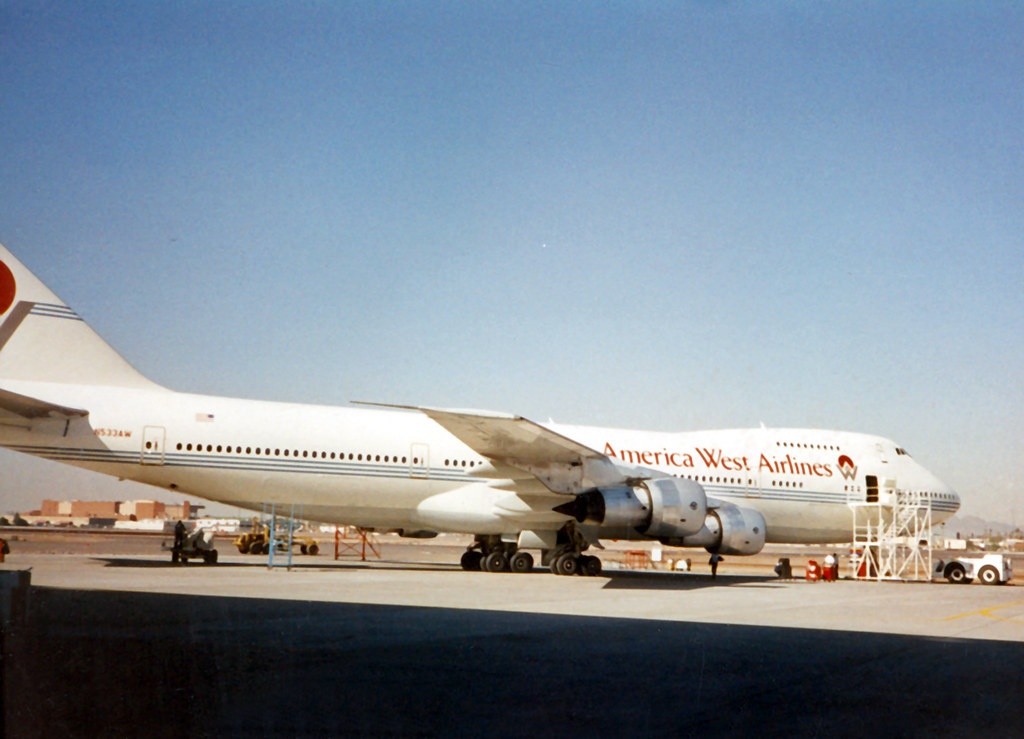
[231,516,320,556]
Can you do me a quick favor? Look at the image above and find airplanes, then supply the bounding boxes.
[0,246,962,574]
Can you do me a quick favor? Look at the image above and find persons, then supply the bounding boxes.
[174,521,187,547]
[833,553,839,579]
[709,554,724,577]
